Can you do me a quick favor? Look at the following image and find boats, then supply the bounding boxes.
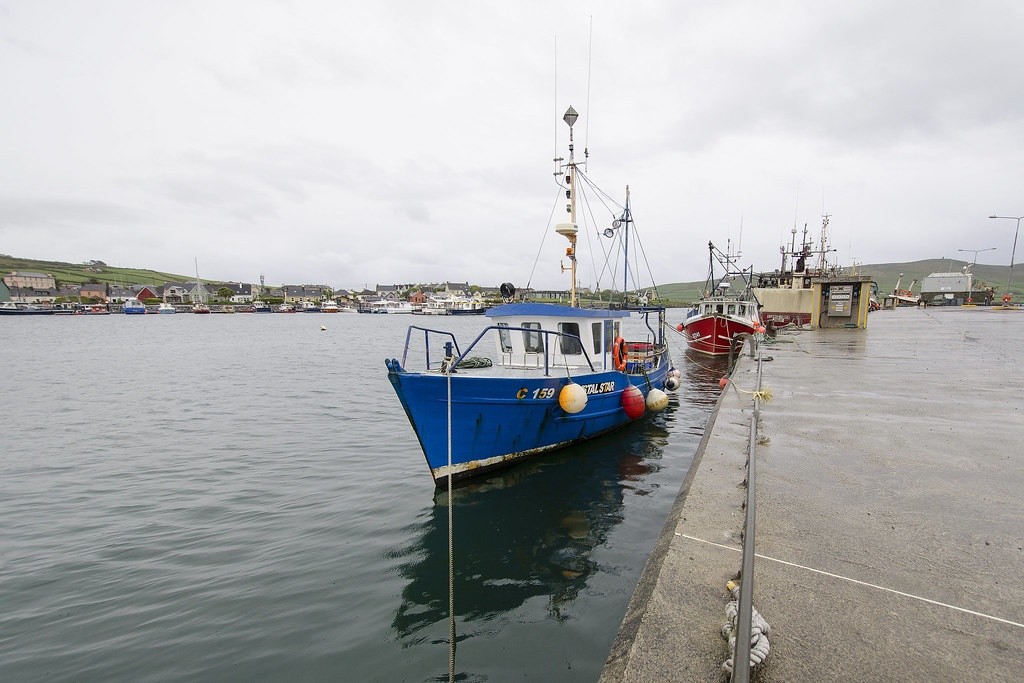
[868,280,881,312]
[190,302,211,313]
[320,292,339,313]
[356,296,372,313]
[888,273,921,306]
[371,300,489,315]
[222,305,235,313]
[683,217,763,355]
[122,298,146,314]
[300,288,321,313]
[0,282,110,315]
[273,291,296,313]
[158,303,176,314]
[751,192,820,327]
[251,293,272,313]
[383,13,681,486]
[238,305,258,312]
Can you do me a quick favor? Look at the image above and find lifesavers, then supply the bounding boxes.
[967,298,972,302]
[614,338,627,370]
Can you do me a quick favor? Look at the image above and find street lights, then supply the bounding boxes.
[957,247,998,278]
[988,215,1024,295]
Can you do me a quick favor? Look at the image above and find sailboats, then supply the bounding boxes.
[590,185,666,312]
